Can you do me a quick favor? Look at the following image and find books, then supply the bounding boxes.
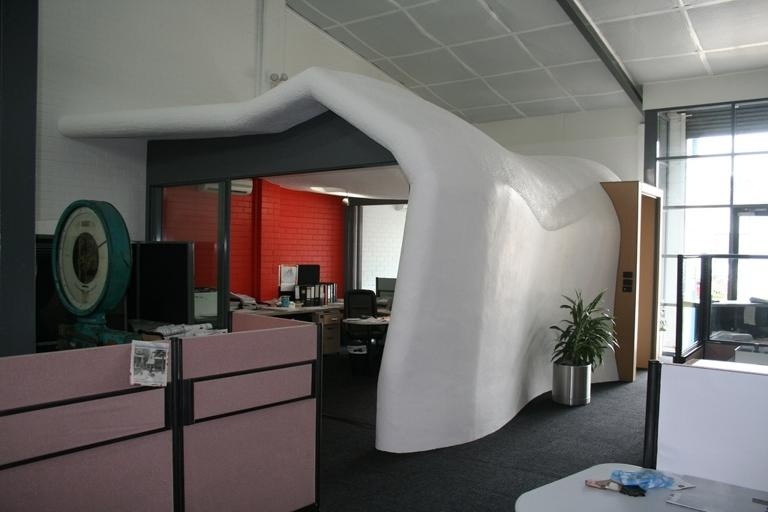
[293,280,338,307]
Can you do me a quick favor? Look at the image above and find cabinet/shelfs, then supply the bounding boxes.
[314,309,341,356]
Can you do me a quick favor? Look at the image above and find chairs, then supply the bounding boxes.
[344,289,388,342]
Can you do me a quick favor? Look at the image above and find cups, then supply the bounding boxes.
[281,296,290,308]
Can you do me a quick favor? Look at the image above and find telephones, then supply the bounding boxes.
[229,292,256,306]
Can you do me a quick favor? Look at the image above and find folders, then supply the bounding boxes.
[294,282,339,306]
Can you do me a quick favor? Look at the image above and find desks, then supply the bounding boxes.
[238,298,345,316]
[342,315,391,359]
[514,461,768,512]
[690,300,768,343]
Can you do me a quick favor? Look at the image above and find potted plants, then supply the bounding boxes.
[549,287,618,406]
